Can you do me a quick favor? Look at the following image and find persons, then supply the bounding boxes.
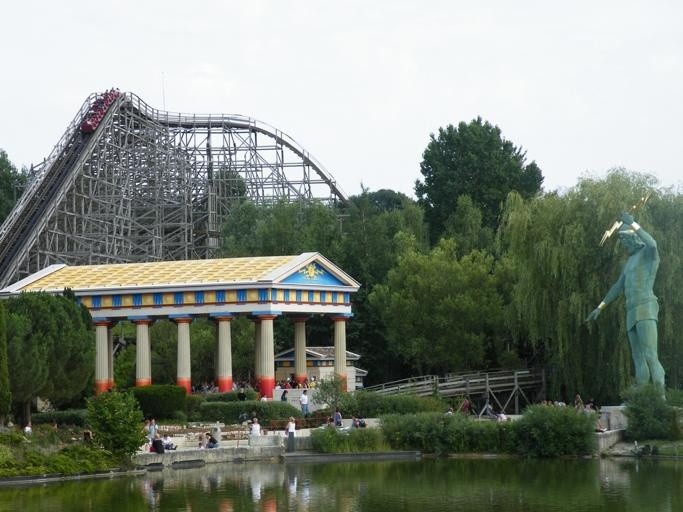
[351,414,367,428]
[298,389,310,416]
[249,417,260,436]
[327,417,335,429]
[460,395,468,413]
[196,432,219,448]
[140,417,177,450]
[23,420,32,436]
[486,400,502,421]
[190,374,320,403]
[327,406,342,425]
[582,212,669,407]
[284,415,295,452]
[539,392,599,413]
